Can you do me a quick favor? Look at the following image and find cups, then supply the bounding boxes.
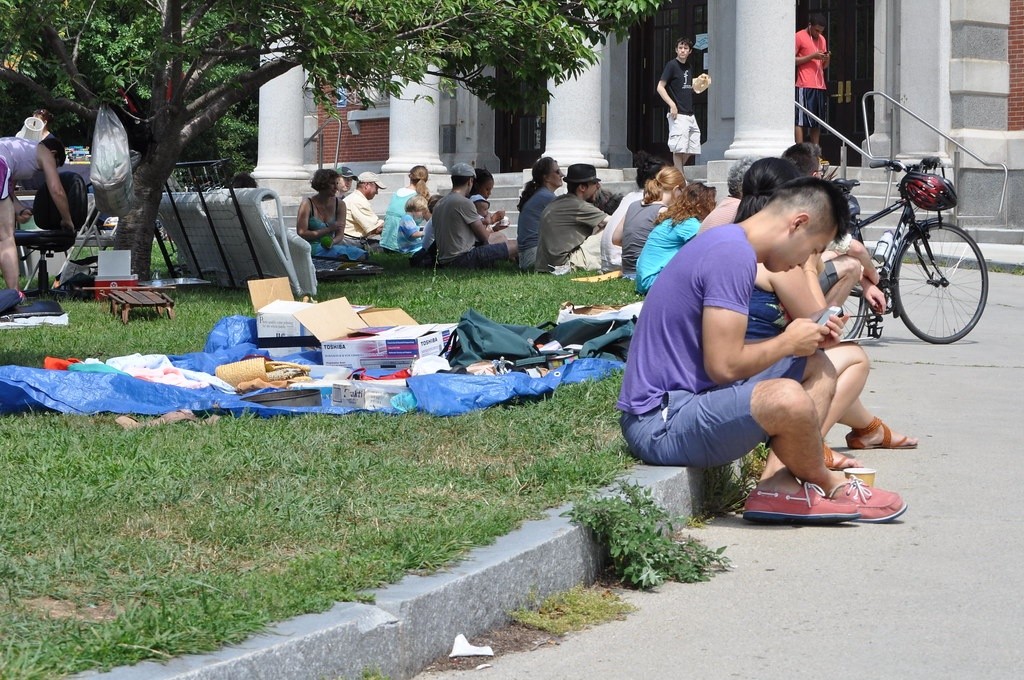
[499,217,510,225]
[843,468,876,488]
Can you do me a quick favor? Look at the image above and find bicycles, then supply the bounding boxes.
[831,157,988,344]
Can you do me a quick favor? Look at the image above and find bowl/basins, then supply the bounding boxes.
[240,389,323,407]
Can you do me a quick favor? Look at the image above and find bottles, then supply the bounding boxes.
[151,269,163,287]
[875,230,893,262]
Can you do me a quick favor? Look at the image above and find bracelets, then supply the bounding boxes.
[804,270,816,275]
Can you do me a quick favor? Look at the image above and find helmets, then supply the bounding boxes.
[904,170,958,211]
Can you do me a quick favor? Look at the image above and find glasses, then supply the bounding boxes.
[547,169,561,178]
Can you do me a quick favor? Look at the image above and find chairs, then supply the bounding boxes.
[75,211,118,260]
[23,192,95,290]
[14,171,88,301]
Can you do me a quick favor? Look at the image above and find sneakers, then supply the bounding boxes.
[823,474,907,522]
[742,482,862,522]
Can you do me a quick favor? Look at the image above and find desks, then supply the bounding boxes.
[57,162,90,186]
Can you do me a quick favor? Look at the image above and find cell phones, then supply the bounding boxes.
[823,51,830,54]
[816,305,843,326]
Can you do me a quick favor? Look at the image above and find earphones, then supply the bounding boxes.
[809,25,811,30]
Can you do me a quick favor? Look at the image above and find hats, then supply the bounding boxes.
[356,172,387,190]
[563,163,599,185]
[451,161,476,179]
[336,166,358,181]
[469,195,490,209]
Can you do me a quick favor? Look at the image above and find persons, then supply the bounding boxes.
[297,169,352,253]
[380,166,433,253]
[785,142,886,316]
[635,182,717,296]
[612,167,687,279]
[601,151,668,274]
[0,137,75,305]
[796,13,830,145]
[33,108,54,139]
[342,171,387,252]
[517,156,564,272]
[423,162,518,270]
[657,37,701,185]
[733,157,919,470]
[335,167,358,199]
[618,176,908,522]
[698,154,764,235]
[397,195,429,254]
[535,164,612,273]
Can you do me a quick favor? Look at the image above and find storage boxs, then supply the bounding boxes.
[94,273,139,299]
[247,276,459,411]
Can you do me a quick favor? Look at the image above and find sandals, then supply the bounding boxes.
[821,440,859,471]
[845,416,918,451]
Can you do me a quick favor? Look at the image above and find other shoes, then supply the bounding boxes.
[17,292,26,302]
